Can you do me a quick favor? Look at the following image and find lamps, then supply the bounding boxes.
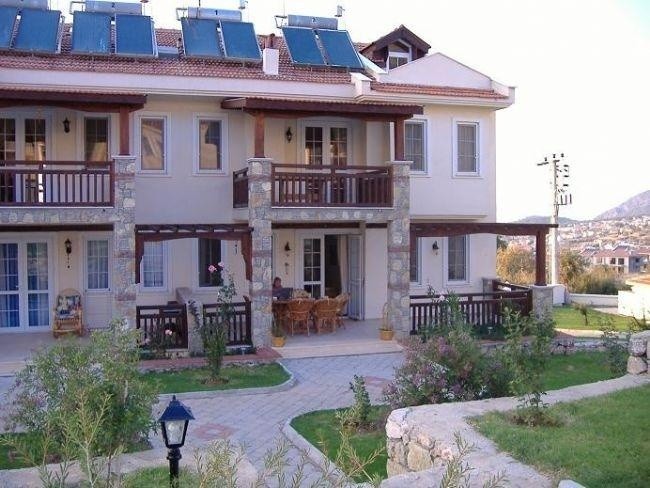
[64,237,72,254]
[62,116,71,133]
[286,126,294,143]
[432,241,439,256]
[284,241,291,257]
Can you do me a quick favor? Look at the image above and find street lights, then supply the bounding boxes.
[156,393,196,487]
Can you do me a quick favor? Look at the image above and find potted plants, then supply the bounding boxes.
[378,301,395,341]
[270,317,286,347]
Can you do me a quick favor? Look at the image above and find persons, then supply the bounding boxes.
[272,277,290,319]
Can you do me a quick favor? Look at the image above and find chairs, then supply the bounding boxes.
[242,288,350,338]
[52,287,84,339]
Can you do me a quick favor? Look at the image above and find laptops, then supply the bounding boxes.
[275,287,293,299]
